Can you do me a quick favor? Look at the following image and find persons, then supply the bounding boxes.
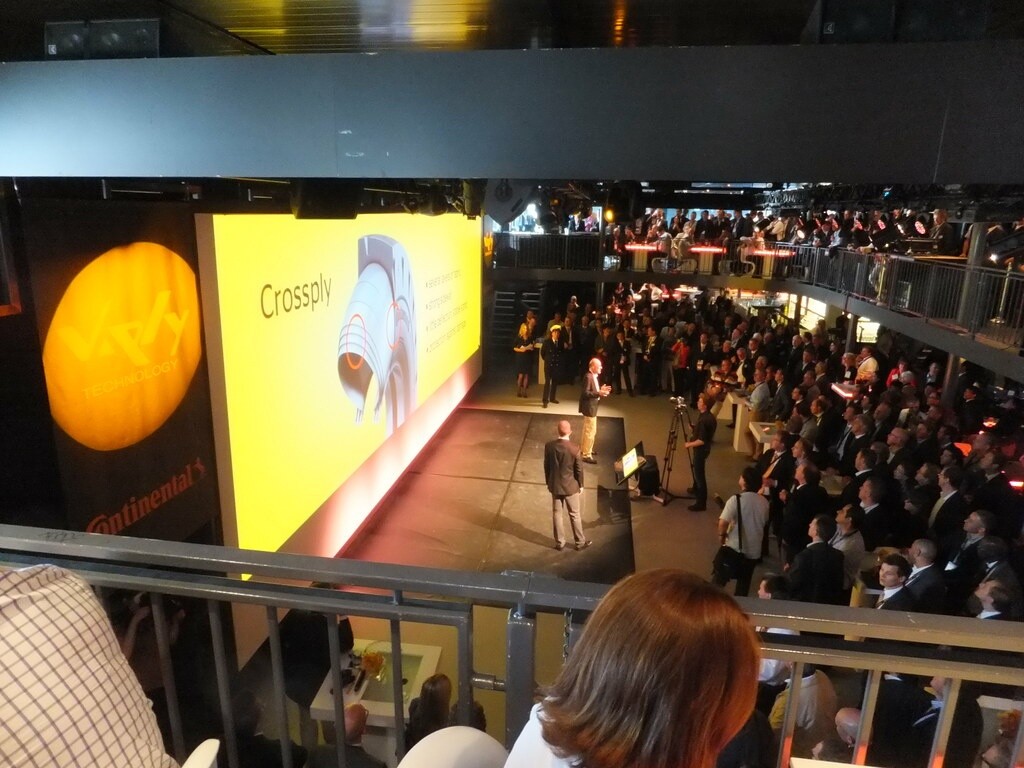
[308,704,388,768]
[685,393,717,511]
[544,420,592,551]
[503,568,762,768]
[514,282,1024,706]
[716,571,984,768]
[217,691,308,768]
[711,466,769,597]
[546,205,1024,355]
[110,579,186,756]
[1,564,180,768]
[281,583,354,750]
[579,357,611,464]
[982,709,1024,768]
[408,673,487,754]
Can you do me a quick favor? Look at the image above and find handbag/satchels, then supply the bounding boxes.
[713,544,753,581]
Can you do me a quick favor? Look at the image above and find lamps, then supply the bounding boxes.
[897,212,917,236]
[832,215,842,230]
[855,213,871,229]
[915,209,934,235]
[816,215,827,227]
[753,219,770,232]
[797,220,817,239]
[877,215,892,230]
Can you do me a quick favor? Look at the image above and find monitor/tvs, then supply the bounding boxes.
[614,440,646,485]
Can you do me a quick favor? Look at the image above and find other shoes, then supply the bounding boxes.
[577,541,592,550]
[522,391,527,397]
[616,390,622,394]
[517,388,521,397]
[543,403,548,408]
[628,391,635,397]
[688,505,706,511]
[687,488,696,494]
[551,399,559,404]
[558,543,567,551]
[726,422,735,428]
[592,450,598,455]
[582,456,598,464]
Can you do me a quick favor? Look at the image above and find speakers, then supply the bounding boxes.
[635,455,660,496]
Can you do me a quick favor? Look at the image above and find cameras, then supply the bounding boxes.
[670,396,687,411]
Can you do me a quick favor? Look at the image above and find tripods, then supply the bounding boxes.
[660,410,696,507]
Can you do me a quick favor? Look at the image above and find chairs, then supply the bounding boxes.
[396,725,509,768]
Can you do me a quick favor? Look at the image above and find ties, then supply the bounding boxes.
[877,592,885,607]
[555,341,557,346]
[758,456,778,494]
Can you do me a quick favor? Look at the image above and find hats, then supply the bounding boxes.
[550,324,562,332]
